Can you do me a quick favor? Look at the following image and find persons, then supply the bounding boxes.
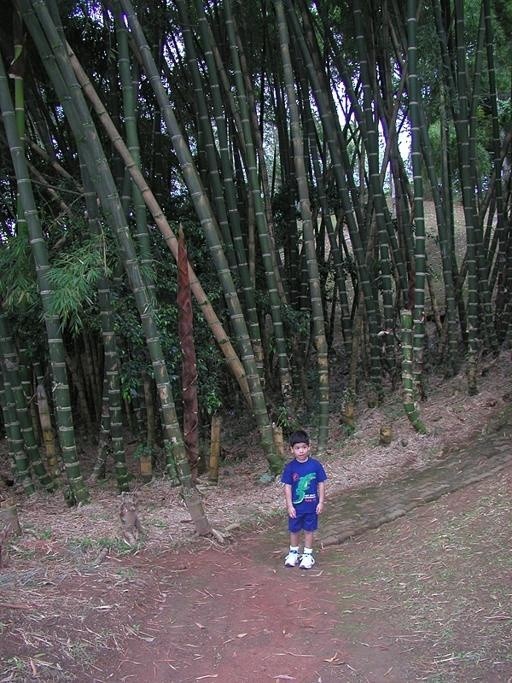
[280,429,327,569]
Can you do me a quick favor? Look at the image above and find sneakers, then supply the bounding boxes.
[300,553,315,569]
[285,550,301,566]
